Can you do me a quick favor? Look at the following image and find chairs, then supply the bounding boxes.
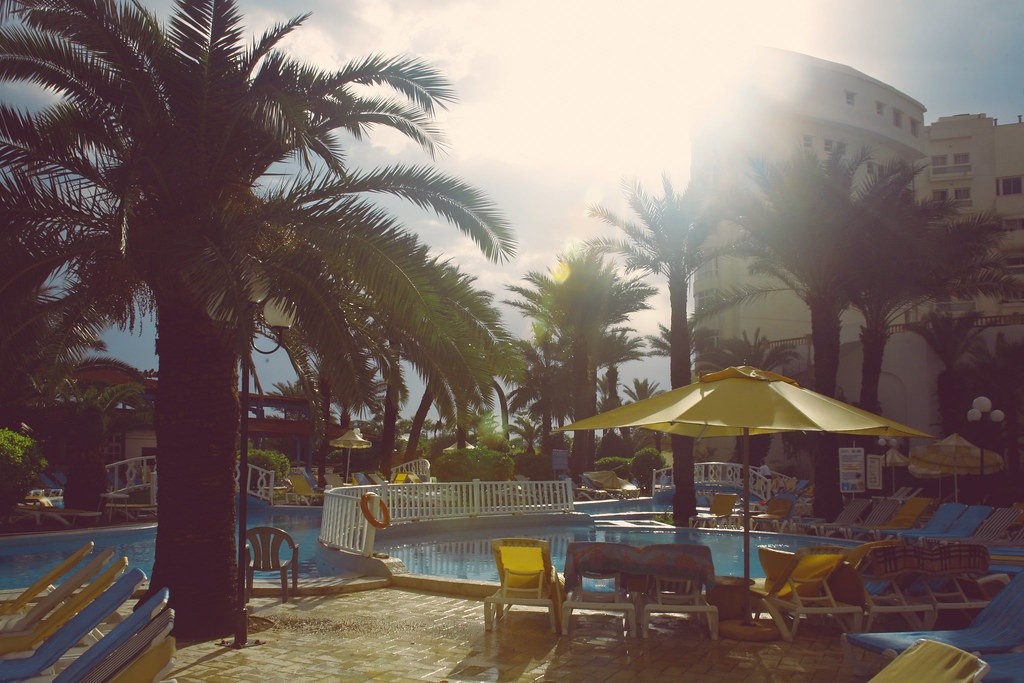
[557,472,641,500]
[284,472,429,507]
[485,491,1024,683]
[0,541,178,683]
[244,526,299,604]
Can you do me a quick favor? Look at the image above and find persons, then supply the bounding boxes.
[759,457,775,499]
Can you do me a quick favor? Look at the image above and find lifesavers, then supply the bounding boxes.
[360,491,390,529]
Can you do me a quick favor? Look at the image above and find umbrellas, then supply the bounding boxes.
[550,357,940,626]
[442,440,482,454]
[329,429,373,483]
[582,470,621,493]
[881,448,908,496]
[908,432,1005,504]
